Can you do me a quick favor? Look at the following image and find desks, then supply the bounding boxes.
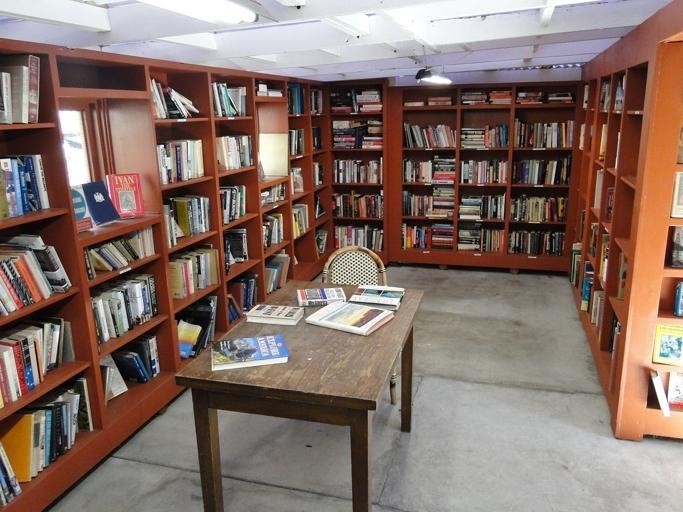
[176,281,424,511]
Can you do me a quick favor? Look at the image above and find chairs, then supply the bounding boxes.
[323,245,401,407]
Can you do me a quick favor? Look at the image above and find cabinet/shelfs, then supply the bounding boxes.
[2,40,181,511]
[570,0,680,441]
[150,59,329,398]
[330,77,576,272]
